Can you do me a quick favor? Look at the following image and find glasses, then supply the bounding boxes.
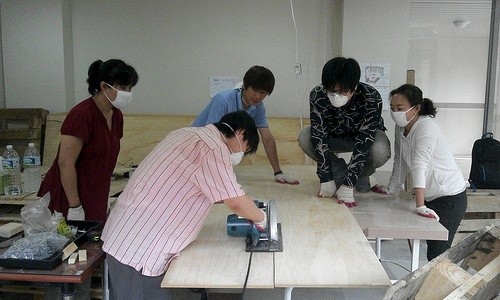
[326,87,352,96]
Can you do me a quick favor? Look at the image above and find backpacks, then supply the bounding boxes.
[468,132,500,190]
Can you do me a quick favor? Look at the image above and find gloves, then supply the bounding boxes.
[67,203,85,221]
[371,184,393,193]
[275,173,299,185]
[318,179,337,198]
[254,209,267,233]
[416,206,440,221]
[336,185,357,207]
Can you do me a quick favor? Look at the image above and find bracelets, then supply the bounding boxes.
[274,171,283,176]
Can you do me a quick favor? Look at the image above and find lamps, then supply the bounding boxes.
[453,21,469,28]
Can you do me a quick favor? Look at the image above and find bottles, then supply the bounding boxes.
[22,143,42,193]
[0,145,23,199]
[57,218,76,243]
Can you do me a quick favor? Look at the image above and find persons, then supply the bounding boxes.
[37,59,139,222]
[371,84,468,262]
[298,57,392,208]
[100,109,267,300]
[191,66,300,185]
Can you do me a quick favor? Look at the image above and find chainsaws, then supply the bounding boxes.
[226,199,283,252]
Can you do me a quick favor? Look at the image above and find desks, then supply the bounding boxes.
[0,234,109,300]
[0,192,42,205]
[349,184,449,284]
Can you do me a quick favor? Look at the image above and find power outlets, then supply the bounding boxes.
[295,64,301,74]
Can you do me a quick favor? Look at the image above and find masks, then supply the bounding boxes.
[326,89,356,107]
[229,137,244,166]
[390,106,416,127]
[103,83,132,109]
